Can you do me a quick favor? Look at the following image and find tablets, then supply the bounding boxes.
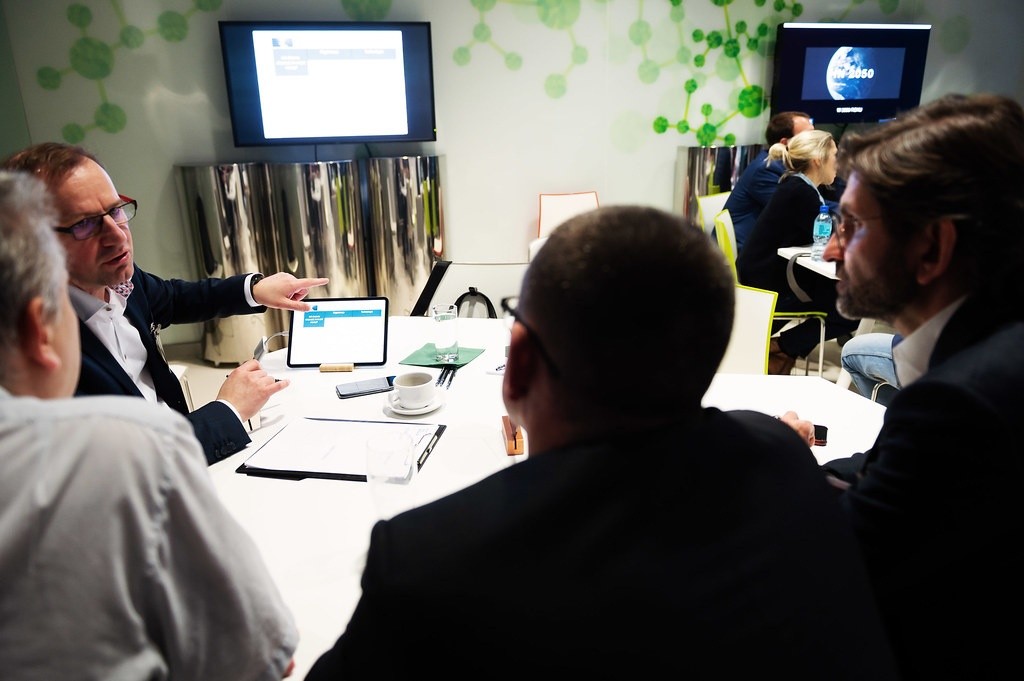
[287,297,390,368]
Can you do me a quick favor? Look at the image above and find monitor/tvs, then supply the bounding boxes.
[218,21,437,148]
[770,24,932,126]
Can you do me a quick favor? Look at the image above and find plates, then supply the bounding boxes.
[386,387,444,415]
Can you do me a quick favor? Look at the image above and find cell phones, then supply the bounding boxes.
[336,375,397,399]
[814,424,827,447]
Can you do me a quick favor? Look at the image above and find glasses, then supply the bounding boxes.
[832,214,967,247]
[502,296,552,375]
[52,194,137,240]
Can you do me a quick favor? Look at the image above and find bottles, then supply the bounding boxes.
[811,206,833,262]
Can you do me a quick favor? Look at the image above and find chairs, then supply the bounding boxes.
[696,192,827,377]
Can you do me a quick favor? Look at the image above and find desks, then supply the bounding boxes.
[777,247,841,284]
[220,316,888,680]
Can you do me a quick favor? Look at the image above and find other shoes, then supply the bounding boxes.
[768,337,796,376]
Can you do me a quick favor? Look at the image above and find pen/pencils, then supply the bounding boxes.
[495,364,505,371]
[435,365,447,386]
[226,375,282,383]
[440,366,451,386]
[446,367,458,389]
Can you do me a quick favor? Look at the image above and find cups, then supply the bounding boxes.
[388,371,434,408]
[432,304,460,363]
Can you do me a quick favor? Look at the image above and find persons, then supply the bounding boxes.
[711,112,861,376]
[769,92,1024,681]
[304,210,901,681]
[0,142,329,466]
[0,171,299,681]
